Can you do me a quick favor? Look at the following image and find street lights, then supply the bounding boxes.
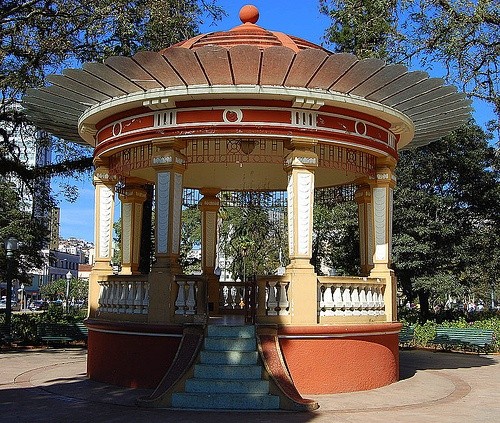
[4,237,15,348]
[20,283,26,311]
[65,271,73,314]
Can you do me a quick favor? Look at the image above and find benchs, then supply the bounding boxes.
[398,326,415,350]
[426,326,495,355]
[38,320,89,349]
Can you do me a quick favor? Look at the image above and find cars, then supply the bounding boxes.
[29,296,84,312]
